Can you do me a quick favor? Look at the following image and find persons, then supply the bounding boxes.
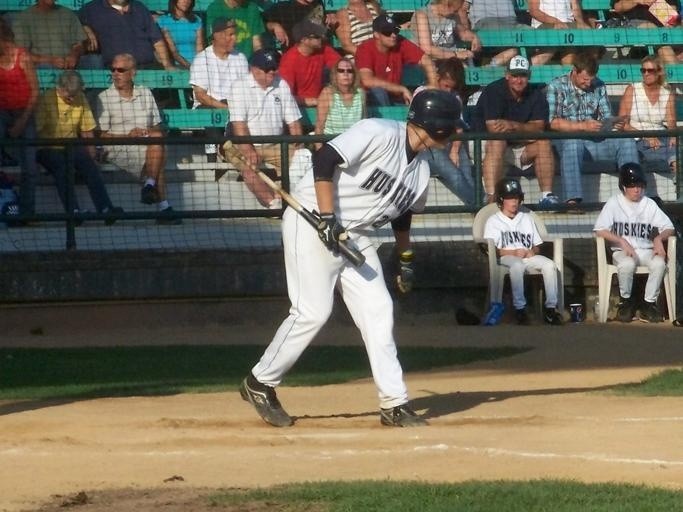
[481,177,563,325]
[591,162,676,323]
[477,56,557,203]
[239,89,461,429]
[0,24,40,227]
[93,54,181,225]
[546,56,639,204]
[618,56,682,203]
[9,0,681,163]
[35,71,122,225]
[219,49,311,209]
[414,57,490,204]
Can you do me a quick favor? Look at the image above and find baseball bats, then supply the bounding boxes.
[223,141,366,267]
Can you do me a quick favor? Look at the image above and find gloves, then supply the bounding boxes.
[395,250,418,294]
[318,215,349,256]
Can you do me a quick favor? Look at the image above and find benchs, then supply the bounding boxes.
[0,0,683,212]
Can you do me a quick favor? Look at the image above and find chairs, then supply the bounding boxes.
[472,201,564,316]
[596,235,676,323]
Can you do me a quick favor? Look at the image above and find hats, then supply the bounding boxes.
[508,56,530,76]
[214,15,402,76]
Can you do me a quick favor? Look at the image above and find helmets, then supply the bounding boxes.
[408,88,470,141]
[619,163,647,192]
[495,179,523,208]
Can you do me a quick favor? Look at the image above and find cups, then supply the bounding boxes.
[569,303,582,322]
[204,142,218,163]
[594,19,606,30]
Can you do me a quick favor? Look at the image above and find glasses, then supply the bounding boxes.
[641,68,657,73]
[110,67,129,72]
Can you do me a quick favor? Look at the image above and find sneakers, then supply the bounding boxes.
[239,372,293,427]
[567,203,583,213]
[516,309,529,325]
[544,310,564,324]
[618,297,633,322]
[72,183,181,225]
[381,406,427,427]
[640,301,664,323]
[539,194,566,213]
